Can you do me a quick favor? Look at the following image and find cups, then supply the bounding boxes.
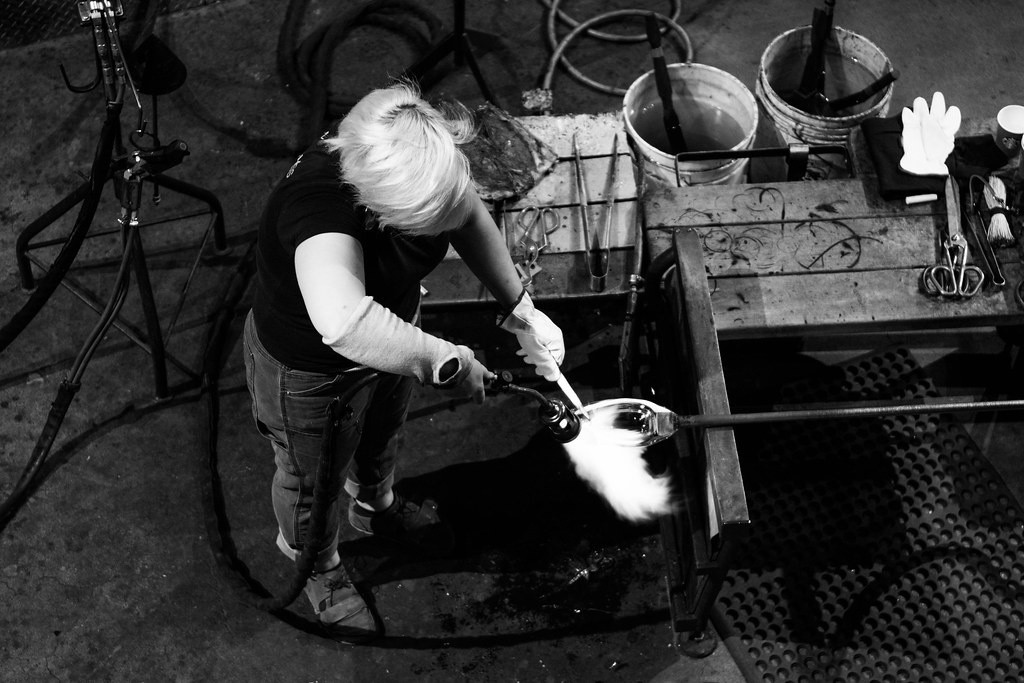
[993,105,1024,159]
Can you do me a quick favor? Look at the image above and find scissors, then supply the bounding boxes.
[919,172,988,298]
[515,204,560,294]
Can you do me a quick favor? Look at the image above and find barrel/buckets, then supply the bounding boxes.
[755,25,895,177]
[622,61,760,185]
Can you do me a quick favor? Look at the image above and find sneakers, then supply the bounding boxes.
[305,561,382,645]
[348,498,443,542]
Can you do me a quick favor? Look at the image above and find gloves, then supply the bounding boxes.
[495,288,566,382]
[898,90,962,177]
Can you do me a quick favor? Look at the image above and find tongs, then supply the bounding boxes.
[571,132,619,293]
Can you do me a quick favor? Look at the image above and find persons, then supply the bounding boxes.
[243,74,566,644]
